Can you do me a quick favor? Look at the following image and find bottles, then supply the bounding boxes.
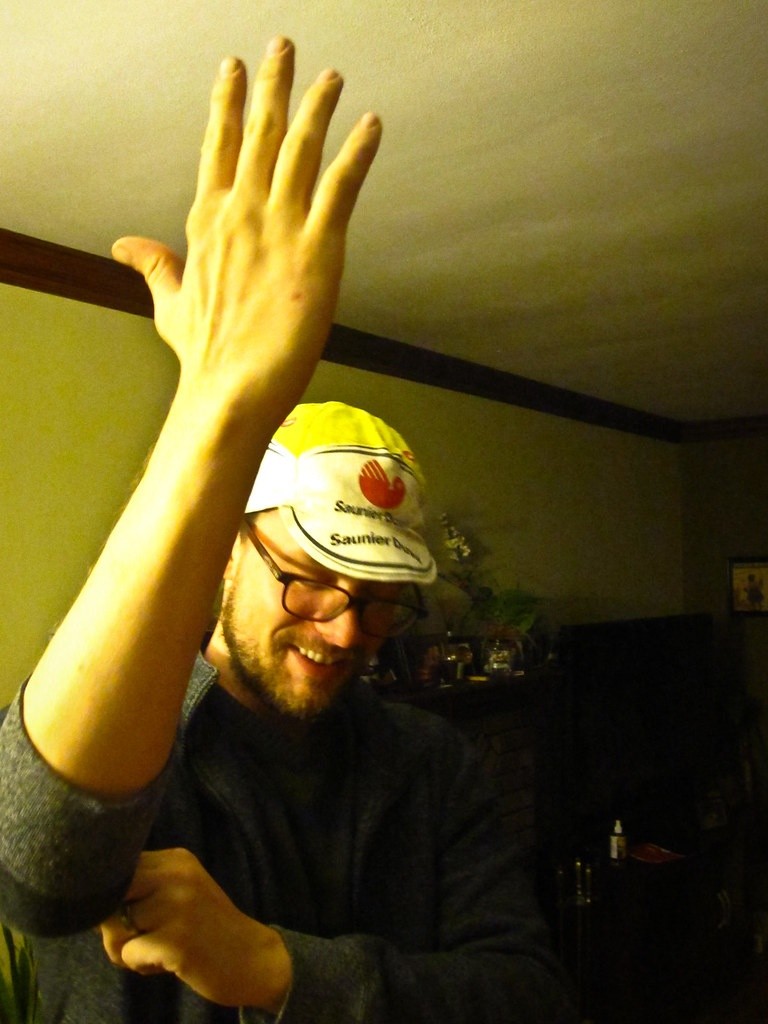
[481,639,527,677]
[610,818,628,860]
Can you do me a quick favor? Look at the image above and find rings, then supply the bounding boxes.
[120,901,144,935]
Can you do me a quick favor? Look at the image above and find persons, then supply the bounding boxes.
[0,37,584,1024]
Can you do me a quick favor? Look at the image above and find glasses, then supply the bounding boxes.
[242,519,429,638]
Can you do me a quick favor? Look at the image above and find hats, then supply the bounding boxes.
[245,402,436,586]
